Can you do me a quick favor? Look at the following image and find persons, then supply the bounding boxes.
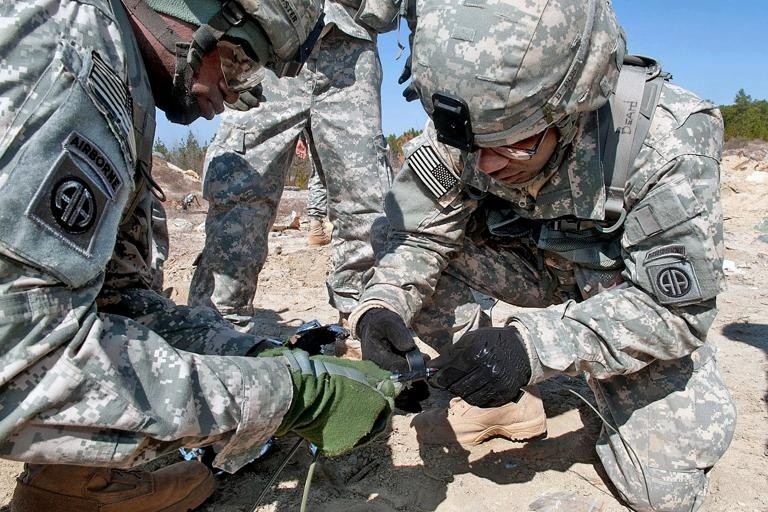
[184,0,406,341]
[348,0,737,510]
[295,124,395,247]
[0,0,404,510]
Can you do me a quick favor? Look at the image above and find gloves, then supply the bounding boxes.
[424,325,530,408]
[224,82,269,113]
[265,320,363,362]
[259,343,401,465]
[358,306,432,384]
[398,13,423,102]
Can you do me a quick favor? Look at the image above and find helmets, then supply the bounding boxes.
[233,0,326,78]
[405,0,629,156]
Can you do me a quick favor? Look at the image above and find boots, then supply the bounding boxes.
[308,220,332,248]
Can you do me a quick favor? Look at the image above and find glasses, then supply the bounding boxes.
[488,124,552,162]
[217,41,266,94]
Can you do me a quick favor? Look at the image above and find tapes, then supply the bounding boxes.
[404,346,425,372]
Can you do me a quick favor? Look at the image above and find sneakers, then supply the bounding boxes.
[407,384,550,450]
[6,459,217,512]
